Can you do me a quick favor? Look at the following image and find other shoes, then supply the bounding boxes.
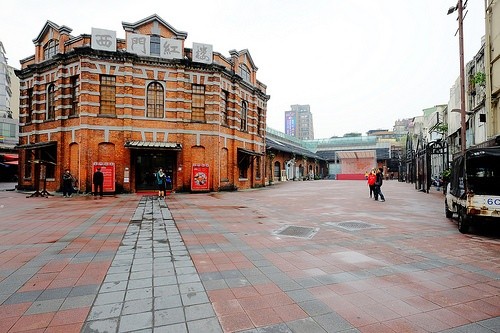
[374,198,378,201]
[162,196,165,199]
[373,196,375,199]
[67,195,73,198]
[379,200,384,202]
[369,196,372,198]
[63,195,67,198]
[158,196,161,200]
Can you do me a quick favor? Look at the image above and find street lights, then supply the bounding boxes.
[446,0,476,153]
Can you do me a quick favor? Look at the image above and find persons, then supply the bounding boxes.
[92,167,104,199]
[62,170,74,198]
[364,167,394,202]
[156,167,167,198]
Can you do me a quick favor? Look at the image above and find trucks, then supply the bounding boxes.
[444,145,500,238]
